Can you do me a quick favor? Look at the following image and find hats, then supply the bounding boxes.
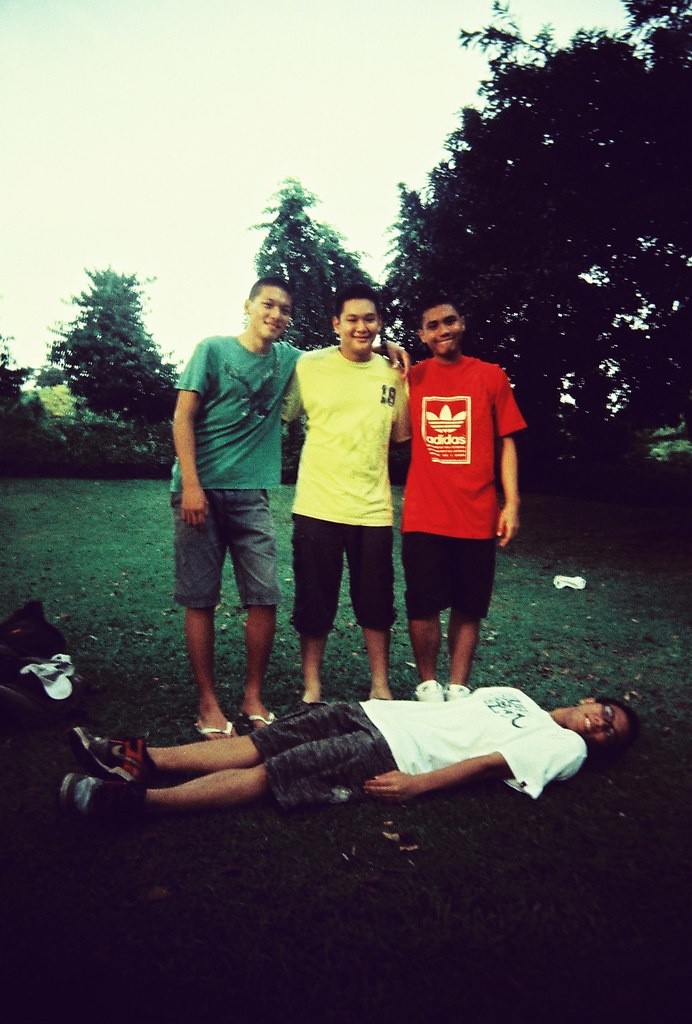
[20,654,74,700]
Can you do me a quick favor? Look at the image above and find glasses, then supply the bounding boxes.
[603,704,618,745]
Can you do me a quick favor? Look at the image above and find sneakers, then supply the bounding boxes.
[70,726,152,783]
[60,772,131,819]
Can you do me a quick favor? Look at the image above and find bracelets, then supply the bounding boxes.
[382,341,386,352]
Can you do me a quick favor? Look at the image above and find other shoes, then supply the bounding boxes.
[416,678,445,702]
[444,683,470,701]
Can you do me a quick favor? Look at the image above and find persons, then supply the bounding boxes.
[59,686,638,821]
[169,277,411,741]
[400,296,528,703]
[282,286,411,713]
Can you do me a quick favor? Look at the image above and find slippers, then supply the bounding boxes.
[239,712,275,730]
[194,721,234,741]
